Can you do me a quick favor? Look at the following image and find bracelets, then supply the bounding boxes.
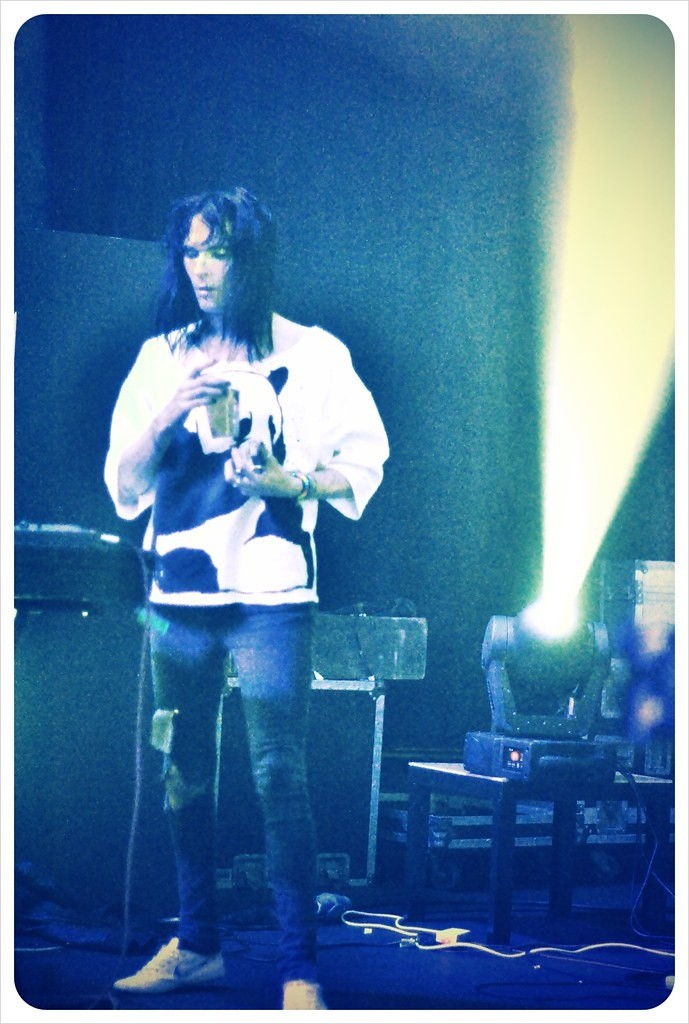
[285,469,318,502]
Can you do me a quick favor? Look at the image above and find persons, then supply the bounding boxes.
[104,183,391,1010]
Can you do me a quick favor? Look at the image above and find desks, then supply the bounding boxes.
[400,759,672,950]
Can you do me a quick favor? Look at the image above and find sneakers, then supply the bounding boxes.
[281,979,326,1010]
[114,936,225,995]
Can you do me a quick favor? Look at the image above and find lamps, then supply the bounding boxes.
[457,611,643,784]
